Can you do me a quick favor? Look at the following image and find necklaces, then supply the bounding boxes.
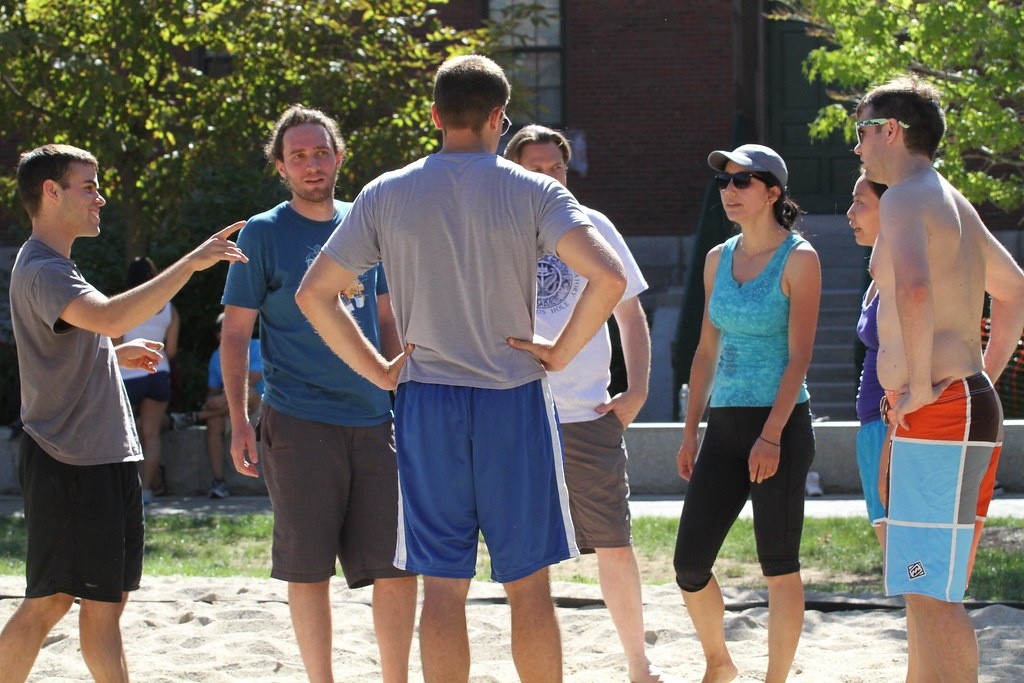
[740,225,783,254]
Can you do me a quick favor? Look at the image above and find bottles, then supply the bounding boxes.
[678,383,688,422]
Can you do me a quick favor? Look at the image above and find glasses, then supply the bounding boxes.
[491,106,512,136]
[855,117,910,144]
[714,171,774,189]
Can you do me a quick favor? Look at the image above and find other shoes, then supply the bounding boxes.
[141,488,150,505]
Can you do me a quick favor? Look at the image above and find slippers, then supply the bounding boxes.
[153,466,168,497]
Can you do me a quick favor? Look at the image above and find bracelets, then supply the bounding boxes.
[758,435,782,446]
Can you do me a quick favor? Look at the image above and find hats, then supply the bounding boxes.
[707,143,788,190]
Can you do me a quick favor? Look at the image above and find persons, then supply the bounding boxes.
[218,102,417,683]
[672,144,821,683]
[171,311,265,497]
[294,56,626,683]
[111,255,185,502]
[504,124,663,683]
[0,142,249,683]
[848,166,890,551]
[857,77,1024,683]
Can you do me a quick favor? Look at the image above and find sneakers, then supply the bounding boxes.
[209,477,230,499]
[170,411,192,433]
